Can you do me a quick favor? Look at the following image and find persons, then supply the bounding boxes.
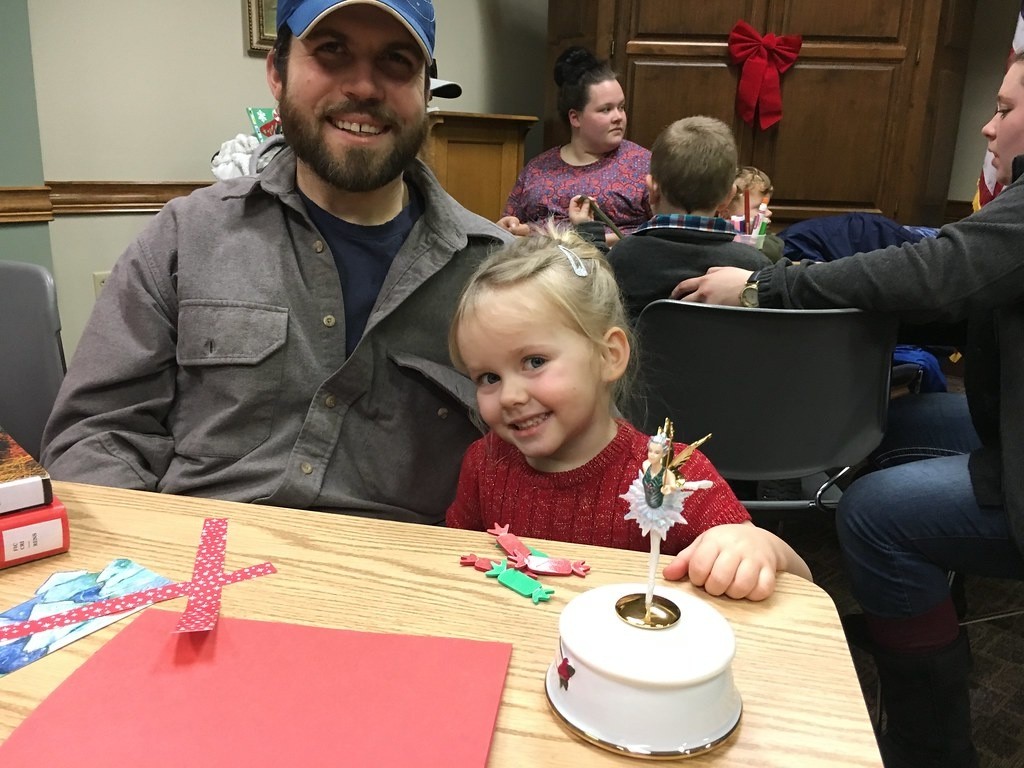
[569,116,774,330]
[495,45,655,249]
[36,0,525,527]
[717,166,785,266]
[447,215,814,602]
[667,50,1023,767]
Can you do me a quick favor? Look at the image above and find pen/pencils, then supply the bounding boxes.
[725,182,769,249]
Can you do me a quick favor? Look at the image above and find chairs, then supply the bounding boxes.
[0,258,67,462]
[626,299,899,513]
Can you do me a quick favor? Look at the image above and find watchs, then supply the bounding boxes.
[739,270,761,308]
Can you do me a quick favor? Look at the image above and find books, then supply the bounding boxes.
[0,426,70,572]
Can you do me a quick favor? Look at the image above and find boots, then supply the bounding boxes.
[877,627,985,766]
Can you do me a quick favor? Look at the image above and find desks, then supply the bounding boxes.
[0,481,885,768]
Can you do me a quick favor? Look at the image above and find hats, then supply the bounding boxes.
[429,78,463,101]
[276,0,437,68]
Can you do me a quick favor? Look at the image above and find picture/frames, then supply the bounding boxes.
[245,0,277,57]
